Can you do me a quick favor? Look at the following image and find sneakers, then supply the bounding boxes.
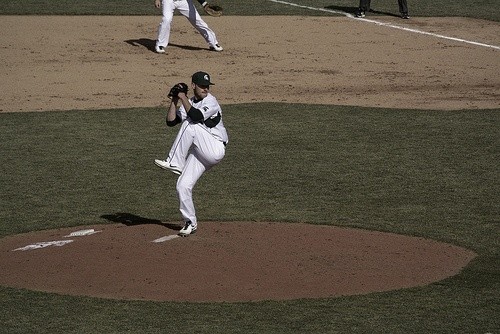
[179,220,198,237]
[154,45,166,53]
[154,157,183,176]
[210,44,223,51]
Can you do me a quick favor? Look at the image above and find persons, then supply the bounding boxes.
[357,0,410,19]
[154,0,223,53]
[154,71,229,236]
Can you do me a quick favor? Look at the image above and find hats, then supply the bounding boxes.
[192,71,215,86]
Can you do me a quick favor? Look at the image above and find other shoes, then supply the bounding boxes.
[401,12,410,19]
[357,7,366,18]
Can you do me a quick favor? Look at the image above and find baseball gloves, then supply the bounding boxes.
[168,82,188,107]
[204,4,225,17]
